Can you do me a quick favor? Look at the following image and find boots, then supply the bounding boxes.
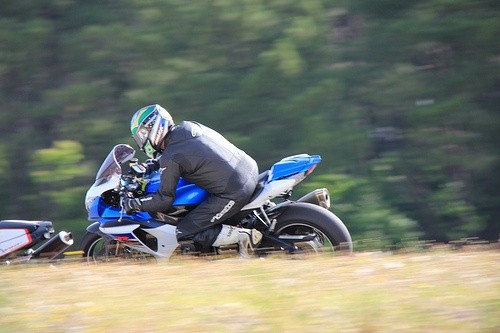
[212,223,263,256]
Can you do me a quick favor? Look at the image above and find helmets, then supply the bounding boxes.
[131,104,175,159]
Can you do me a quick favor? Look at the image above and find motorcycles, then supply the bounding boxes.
[80,144,354,264]
[0,219,75,266]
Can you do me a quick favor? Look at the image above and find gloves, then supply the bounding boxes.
[123,197,143,213]
[145,159,158,170]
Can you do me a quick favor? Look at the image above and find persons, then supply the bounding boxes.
[123,104,264,257]
